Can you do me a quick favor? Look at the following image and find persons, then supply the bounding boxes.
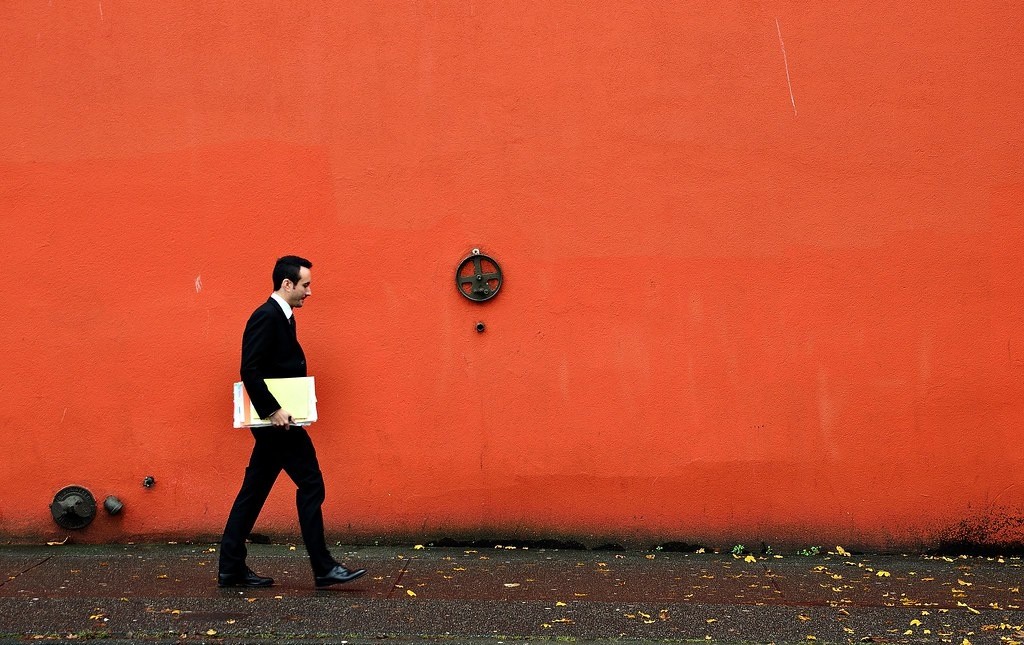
[218,255,369,586]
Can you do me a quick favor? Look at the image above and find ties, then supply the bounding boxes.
[289,314,296,338]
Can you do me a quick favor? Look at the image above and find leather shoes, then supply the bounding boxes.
[314,562,367,587]
[217,566,275,587]
